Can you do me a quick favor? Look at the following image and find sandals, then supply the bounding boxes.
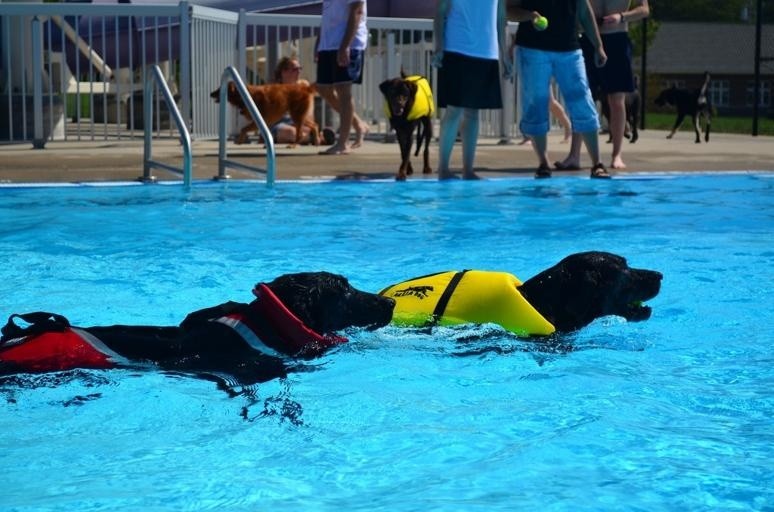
[533,162,552,179]
[589,162,612,179]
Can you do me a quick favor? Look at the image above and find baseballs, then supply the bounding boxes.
[534,16,548,30]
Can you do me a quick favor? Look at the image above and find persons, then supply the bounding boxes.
[259,0,369,154]
[430,0,650,179]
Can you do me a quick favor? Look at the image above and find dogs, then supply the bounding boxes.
[379,247,664,369]
[378,63,436,181]
[1,271,397,428]
[653,69,715,144]
[598,73,645,143]
[209,76,322,149]
